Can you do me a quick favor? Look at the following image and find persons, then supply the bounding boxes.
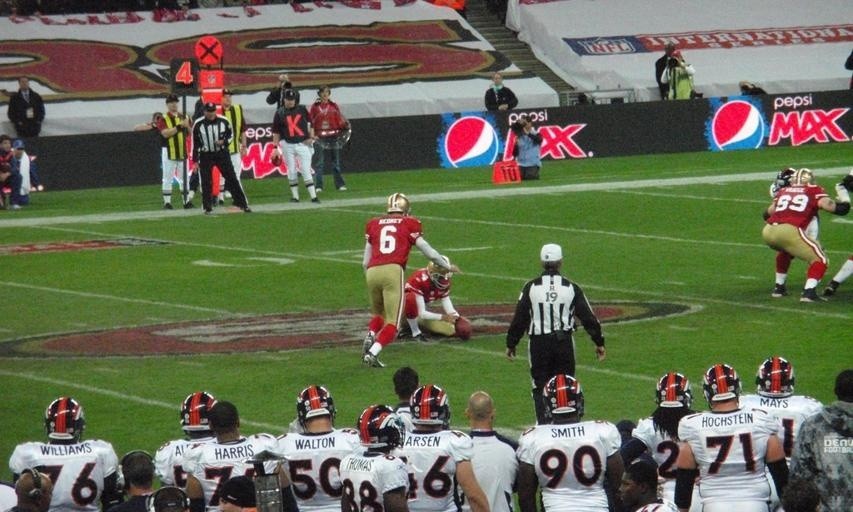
[359,193,464,368]
[739,81,766,94]
[340,368,519,512]
[10,140,29,203]
[761,169,853,303]
[484,74,518,110]
[515,373,697,512]
[661,51,696,100]
[576,94,589,104]
[106,450,191,512]
[397,255,460,344]
[505,244,606,426]
[0,135,23,209]
[153,385,360,512]
[8,397,125,512]
[655,41,675,100]
[265,74,349,202]
[513,116,543,179]
[7,76,45,136]
[135,88,252,213]
[677,357,852,512]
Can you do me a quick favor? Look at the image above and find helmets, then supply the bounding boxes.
[357,403,407,450]
[655,370,694,408]
[43,396,86,438]
[425,256,452,289]
[703,362,742,400]
[409,384,451,427]
[755,355,797,393]
[385,193,412,215]
[543,374,585,415]
[775,167,814,187]
[294,384,336,428]
[179,390,219,434]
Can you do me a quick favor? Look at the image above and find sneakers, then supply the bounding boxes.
[397,324,428,344]
[771,282,792,298]
[164,185,250,216]
[361,331,386,367]
[292,182,348,205]
[821,286,834,301]
[800,286,823,303]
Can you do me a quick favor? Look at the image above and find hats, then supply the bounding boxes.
[203,101,216,113]
[165,94,180,104]
[283,89,296,101]
[223,89,234,96]
[670,50,681,58]
[13,139,26,152]
[540,243,563,265]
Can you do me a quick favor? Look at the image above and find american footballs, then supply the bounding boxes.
[455,316,471,340]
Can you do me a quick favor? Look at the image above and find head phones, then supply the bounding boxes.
[115,448,153,495]
[21,467,43,499]
